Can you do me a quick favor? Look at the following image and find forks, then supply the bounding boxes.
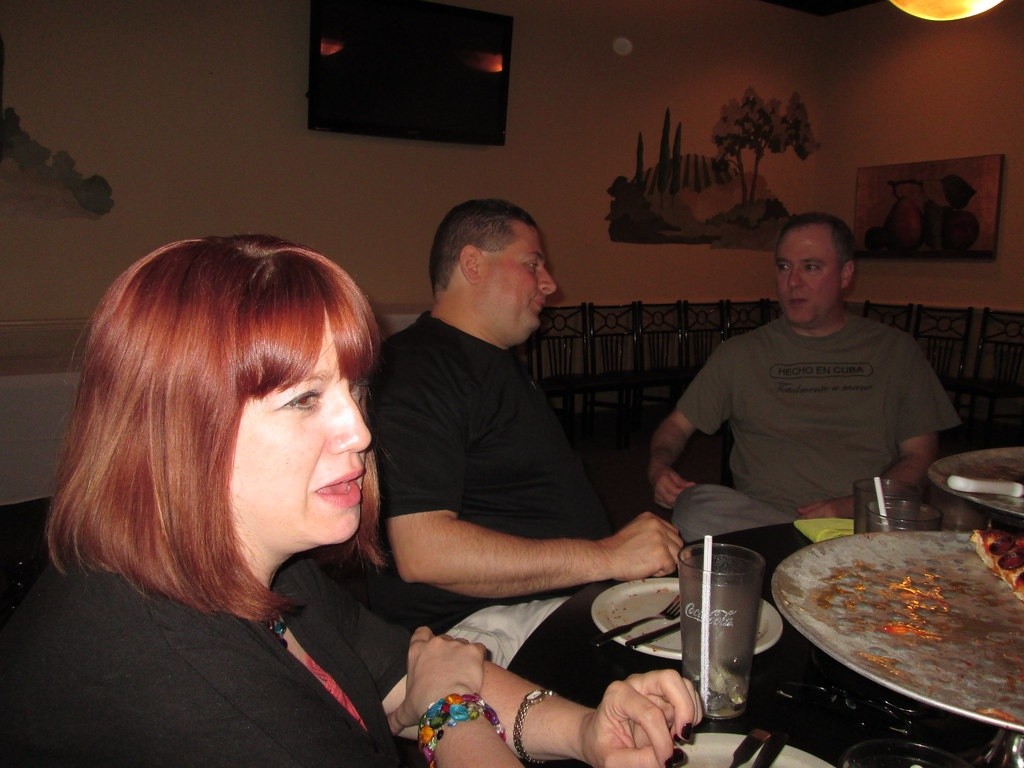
[593,595,680,646]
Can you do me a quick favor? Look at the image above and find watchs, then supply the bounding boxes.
[511,688,564,764]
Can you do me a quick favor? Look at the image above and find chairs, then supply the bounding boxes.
[514,298,1024,473]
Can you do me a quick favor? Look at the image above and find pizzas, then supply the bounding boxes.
[969,530,1024,601]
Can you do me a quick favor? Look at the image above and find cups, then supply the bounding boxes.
[839,740,974,768]
[678,543,765,719]
[854,479,923,534]
[865,501,943,533]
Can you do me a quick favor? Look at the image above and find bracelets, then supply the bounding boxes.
[414,692,509,764]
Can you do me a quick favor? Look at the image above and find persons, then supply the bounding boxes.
[640,211,965,542]
[0,236,702,768]
[359,199,689,645]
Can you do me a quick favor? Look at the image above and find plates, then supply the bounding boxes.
[666,733,835,768]
[591,578,783,660]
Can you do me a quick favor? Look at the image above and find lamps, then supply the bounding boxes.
[890,0,1002,21]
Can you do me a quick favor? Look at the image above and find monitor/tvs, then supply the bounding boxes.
[306,0,514,146]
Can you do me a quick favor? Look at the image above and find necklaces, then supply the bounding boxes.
[268,614,290,646]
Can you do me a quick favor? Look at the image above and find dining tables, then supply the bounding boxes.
[503,504,1024,768]
